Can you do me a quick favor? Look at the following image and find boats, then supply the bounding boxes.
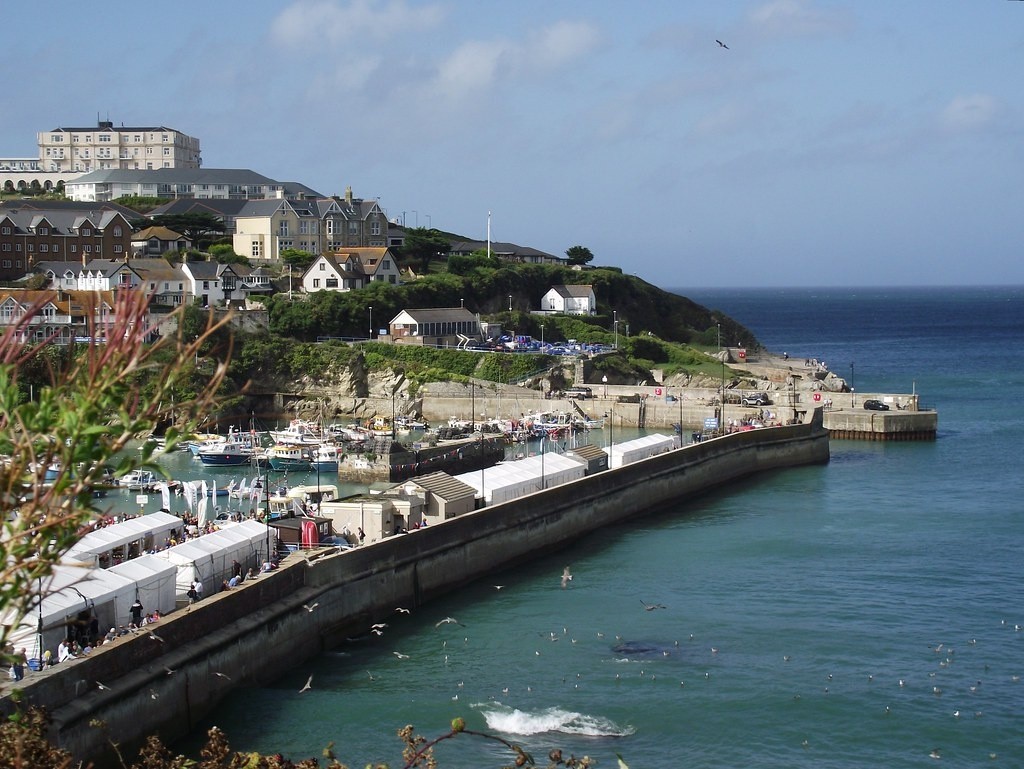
[0,368,606,517]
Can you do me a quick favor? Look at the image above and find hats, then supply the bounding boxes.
[110,628,115,632]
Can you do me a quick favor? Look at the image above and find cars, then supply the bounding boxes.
[863,399,890,411]
[465,334,611,356]
[722,393,741,404]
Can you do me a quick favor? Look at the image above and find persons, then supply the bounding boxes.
[187,578,203,604]
[675,423,681,433]
[805,357,825,372]
[100,511,220,565]
[343,526,350,538]
[220,548,282,592]
[691,430,701,444]
[824,398,832,408]
[12,599,164,681]
[394,519,427,535]
[358,527,364,544]
[78,507,143,532]
[301,501,314,516]
[547,390,562,400]
[896,401,909,410]
[230,509,266,523]
[728,409,771,427]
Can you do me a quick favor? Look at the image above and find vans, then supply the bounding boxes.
[562,386,592,398]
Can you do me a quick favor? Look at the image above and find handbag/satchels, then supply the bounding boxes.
[9,667,17,678]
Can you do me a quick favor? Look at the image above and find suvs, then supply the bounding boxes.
[742,391,768,406]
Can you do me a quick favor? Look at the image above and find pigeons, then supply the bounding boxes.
[146,628,164,642]
[371,623,389,628]
[442,619,1023,769]
[640,597,667,612]
[299,676,313,694]
[494,585,504,590]
[367,670,375,681]
[96,680,112,692]
[149,688,159,700]
[560,566,572,589]
[435,617,466,628]
[164,667,177,675]
[210,672,231,681]
[371,629,383,636]
[393,652,410,659]
[395,607,410,615]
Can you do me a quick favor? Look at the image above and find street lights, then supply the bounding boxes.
[613,310,616,333]
[384,386,405,440]
[672,392,683,447]
[534,424,544,490]
[614,320,619,348]
[474,434,485,509]
[368,306,373,340]
[31,575,44,672]
[460,298,464,308]
[790,374,797,424]
[717,323,721,350]
[305,451,320,517]
[540,324,545,354]
[194,334,200,365]
[848,361,855,408]
[253,472,271,562]
[509,295,513,313]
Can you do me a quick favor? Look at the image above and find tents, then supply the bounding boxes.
[602,433,674,469]
[156,520,275,601]
[0,554,178,667]
[56,510,184,570]
[454,452,585,507]
